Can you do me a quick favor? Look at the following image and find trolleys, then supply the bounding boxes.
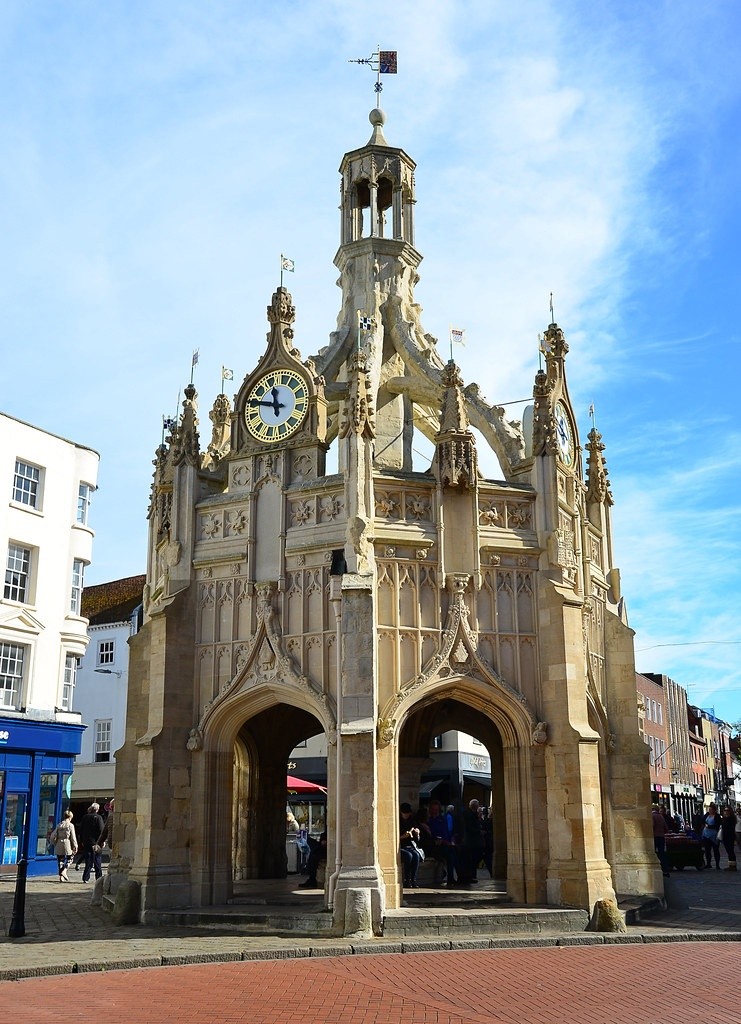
[665,830,706,873]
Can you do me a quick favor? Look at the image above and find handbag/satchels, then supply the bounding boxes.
[717,827,722,840]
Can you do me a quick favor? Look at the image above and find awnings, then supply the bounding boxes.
[672,783,698,798]
[463,774,491,792]
[417,779,445,797]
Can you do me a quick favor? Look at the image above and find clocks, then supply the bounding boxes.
[244,368,310,443]
[555,401,575,469]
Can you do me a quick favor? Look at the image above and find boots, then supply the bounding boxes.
[724,861,737,871]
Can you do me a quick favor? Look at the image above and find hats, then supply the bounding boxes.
[652,804,659,810]
[400,803,412,813]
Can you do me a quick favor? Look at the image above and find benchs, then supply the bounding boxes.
[102,894,116,908]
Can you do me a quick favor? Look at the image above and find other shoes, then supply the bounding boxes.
[716,866,720,869]
[299,879,317,887]
[84,881,89,883]
[76,865,80,871]
[704,864,712,869]
[470,879,478,883]
[61,868,69,881]
[405,881,413,887]
[60,876,66,881]
[412,882,419,887]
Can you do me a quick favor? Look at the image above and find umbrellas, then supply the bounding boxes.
[287,775,328,793]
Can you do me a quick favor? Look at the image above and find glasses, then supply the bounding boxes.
[724,809,731,811]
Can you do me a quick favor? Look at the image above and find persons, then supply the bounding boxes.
[692,804,706,871]
[93,797,114,854]
[298,830,327,889]
[735,808,741,850]
[651,804,675,877]
[50,810,79,882]
[75,802,105,882]
[699,803,722,870]
[721,805,738,872]
[400,799,493,888]
